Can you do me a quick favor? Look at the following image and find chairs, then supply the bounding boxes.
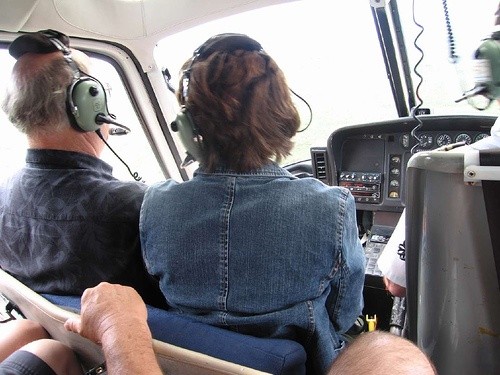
[406,150,500,375]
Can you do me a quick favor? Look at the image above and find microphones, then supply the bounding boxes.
[103,118,131,136]
[455,91,478,103]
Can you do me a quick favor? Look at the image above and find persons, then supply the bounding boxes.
[377,116,500,297]
[0,282,435,375]
[0,47,171,311]
[139,33,367,375]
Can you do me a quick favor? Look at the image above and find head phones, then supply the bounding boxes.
[473,4,500,101]
[170,32,264,163]
[7,29,109,133]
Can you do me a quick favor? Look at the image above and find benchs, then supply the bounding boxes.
[0,267,305,375]
